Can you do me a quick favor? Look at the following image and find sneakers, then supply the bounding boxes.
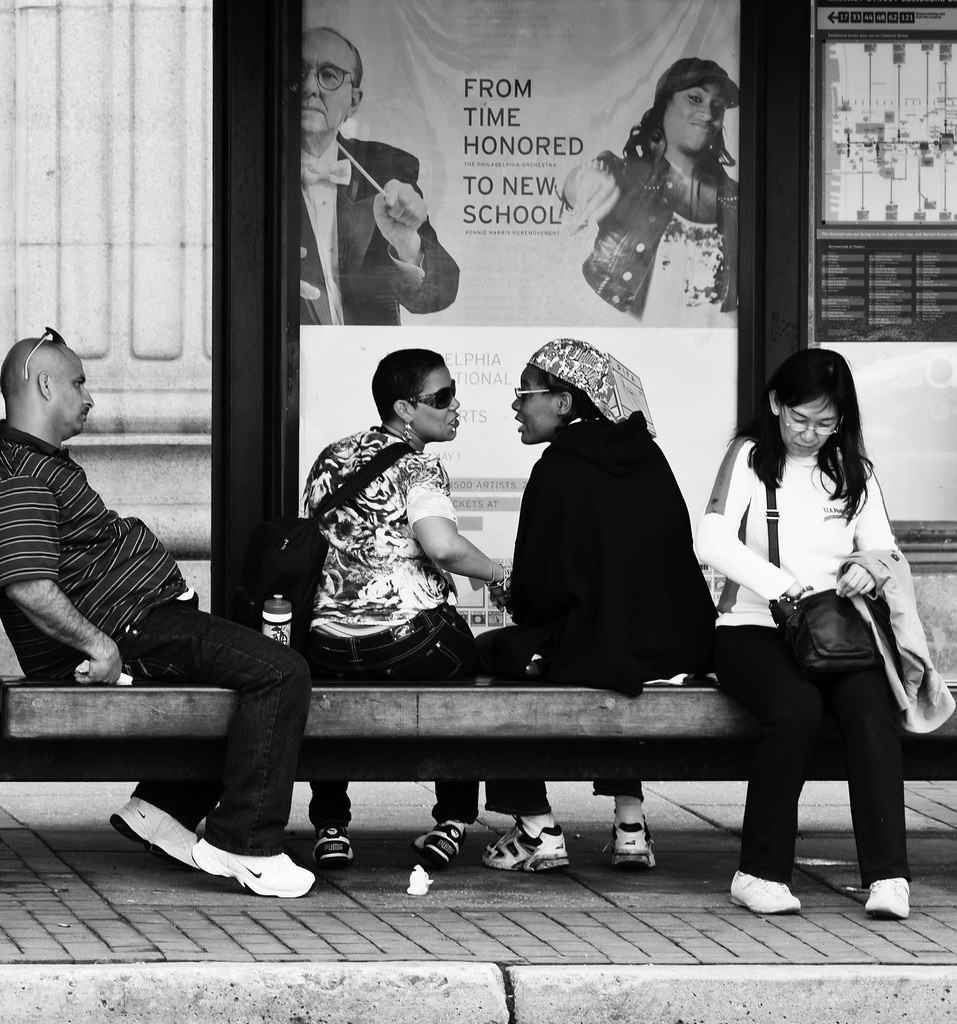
[414,822,466,865]
[865,873,910,919]
[482,813,570,871]
[190,834,316,898]
[311,824,353,862]
[728,868,800,914]
[601,806,657,867]
[109,795,202,868]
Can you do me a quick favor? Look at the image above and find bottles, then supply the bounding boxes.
[262,593,293,648]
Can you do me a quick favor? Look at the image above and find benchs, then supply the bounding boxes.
[0,675,957,779]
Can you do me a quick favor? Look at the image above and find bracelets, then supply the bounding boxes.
[484,557,496,585]
[794,585,814,599]
[498,562,511,590]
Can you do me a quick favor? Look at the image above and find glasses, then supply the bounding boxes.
[300,60,356,91]
[779,399,845,436]
[513,387,552,406]
[409,379,456,410]
[23,326,66,381]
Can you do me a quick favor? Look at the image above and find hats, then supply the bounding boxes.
[529,338,615,422]
[655,58,739,110]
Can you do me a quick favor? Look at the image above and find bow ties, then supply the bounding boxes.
[302,156,352,187]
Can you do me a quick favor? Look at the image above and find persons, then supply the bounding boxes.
[483,339,718,872]
[1,327,316,897]
[304,347,503,866]
[560,59,738,322]
[695,349,955,919]
[296,27,461,324]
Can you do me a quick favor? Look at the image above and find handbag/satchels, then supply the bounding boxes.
[227,513,330,655]
[770,585,885,677]
